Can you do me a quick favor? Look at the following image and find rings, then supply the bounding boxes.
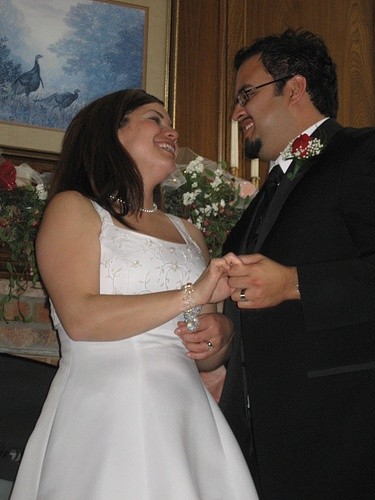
[240,288,248,302]
[205,341,213,350]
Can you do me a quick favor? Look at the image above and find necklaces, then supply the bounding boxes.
[109,195,158,212]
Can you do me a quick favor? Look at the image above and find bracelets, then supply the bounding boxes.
[180,283,200,330]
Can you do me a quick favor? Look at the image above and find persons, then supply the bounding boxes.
[11,88,258,500]
[218,27,375,500]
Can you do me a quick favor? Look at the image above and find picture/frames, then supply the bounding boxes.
[0,0,173,171]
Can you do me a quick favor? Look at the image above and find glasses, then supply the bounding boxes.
[231,73,296,111]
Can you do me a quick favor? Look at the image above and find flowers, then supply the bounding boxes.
[160,154,257,259]
[279,132,323,174]
[0,183,50,324]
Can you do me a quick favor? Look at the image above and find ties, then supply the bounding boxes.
[245,166,285,254]
